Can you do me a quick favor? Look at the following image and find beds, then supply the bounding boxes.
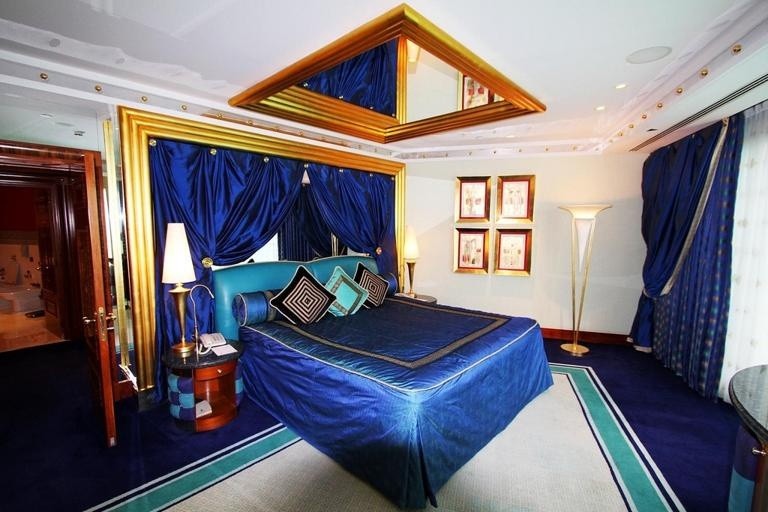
[211,254,544,512]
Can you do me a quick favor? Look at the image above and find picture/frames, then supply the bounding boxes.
[453,175,536,276]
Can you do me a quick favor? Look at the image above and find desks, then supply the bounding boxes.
[729,362,768,512]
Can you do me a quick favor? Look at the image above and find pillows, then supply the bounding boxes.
[231,261,398,328]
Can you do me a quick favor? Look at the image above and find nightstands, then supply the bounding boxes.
[160,339,246,434]
[401,293,437,305]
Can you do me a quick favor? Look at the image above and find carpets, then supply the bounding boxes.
[86,360,688,511]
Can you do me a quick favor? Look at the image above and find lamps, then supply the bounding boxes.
[162,223,197,354]
[556,203,613,357]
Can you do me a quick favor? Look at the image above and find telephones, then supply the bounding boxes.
[200,332,226,348]
